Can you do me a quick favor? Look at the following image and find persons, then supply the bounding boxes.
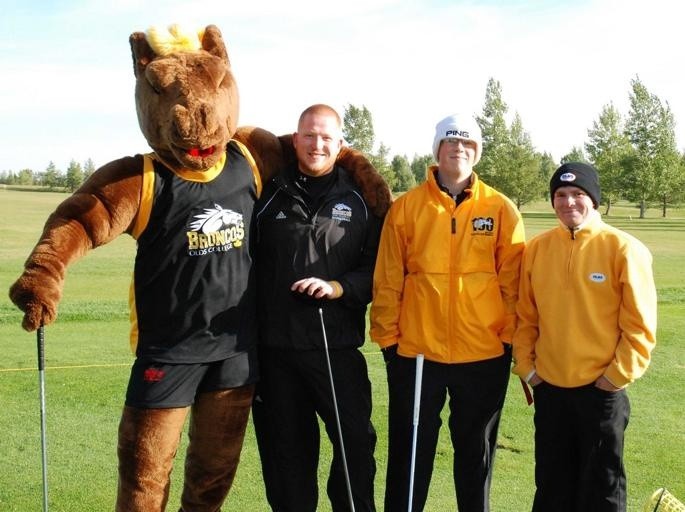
[514,161,660,511]
[368,111,529,511]
[248,103,380,511]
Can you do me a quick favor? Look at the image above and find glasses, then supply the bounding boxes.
[442,137,477,148]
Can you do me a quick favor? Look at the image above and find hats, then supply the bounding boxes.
[549,161,603,212]
[431,112,485,169]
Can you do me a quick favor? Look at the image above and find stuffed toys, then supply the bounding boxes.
[6,19,397,511]
